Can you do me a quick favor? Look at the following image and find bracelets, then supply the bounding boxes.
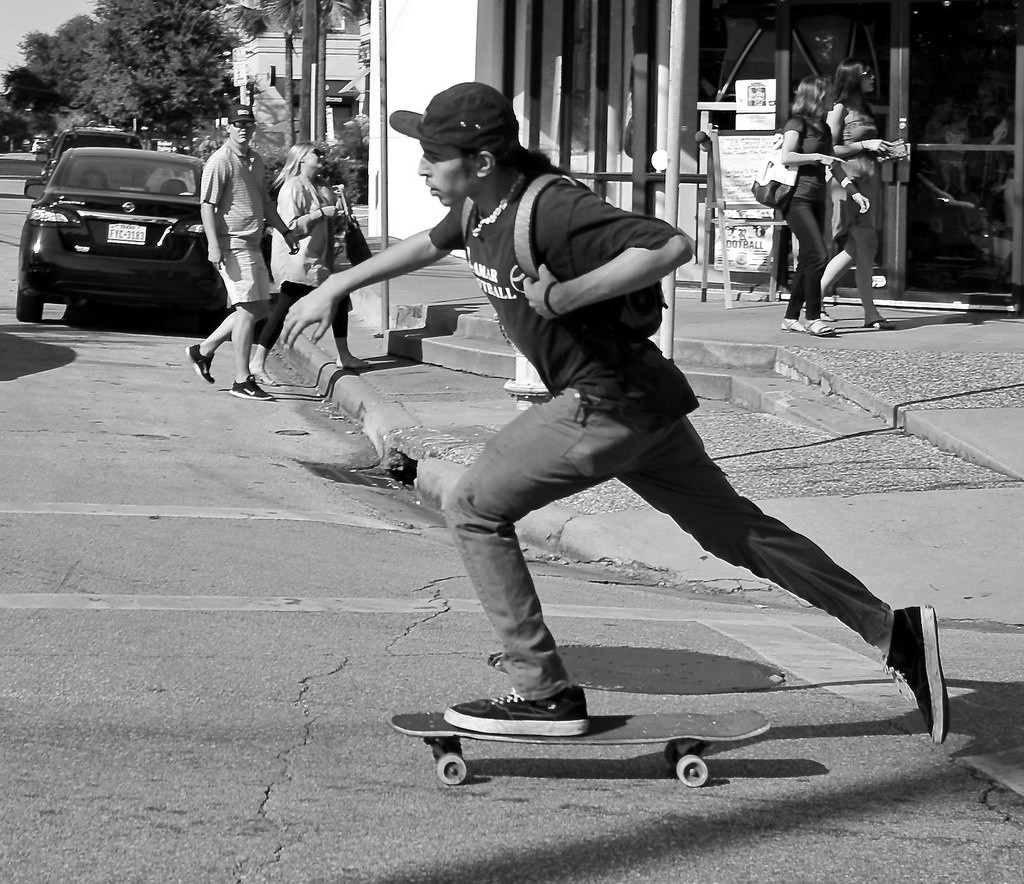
[859,141,864,149]
[543,282,563,317]
[282,229,292,237]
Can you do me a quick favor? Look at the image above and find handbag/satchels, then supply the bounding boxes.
[336,185,371,266]
[751,115,808,213]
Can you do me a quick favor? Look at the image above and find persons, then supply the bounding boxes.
[185,104,300,401]
[249,141,374,387]
[825,56,897,330]
[775,75,871,337]
[279,82,951,746]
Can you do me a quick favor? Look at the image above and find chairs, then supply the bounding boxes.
[159,177,189,196]
[78,168,110,190]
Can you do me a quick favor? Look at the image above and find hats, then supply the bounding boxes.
[389,83,521,158]
[227,104,258,124]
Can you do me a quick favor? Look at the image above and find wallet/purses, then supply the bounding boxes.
[876,138,908,164]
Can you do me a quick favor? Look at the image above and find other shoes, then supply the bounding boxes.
[929,216,943,233]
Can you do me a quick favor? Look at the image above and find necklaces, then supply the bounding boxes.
[468,174,524,237]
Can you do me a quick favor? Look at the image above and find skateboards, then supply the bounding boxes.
[387,708,773,788]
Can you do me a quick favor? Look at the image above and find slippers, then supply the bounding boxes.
[819,311,837,322]
[251,371,277,387]
[860,319,896,330]
[336,359,375,370]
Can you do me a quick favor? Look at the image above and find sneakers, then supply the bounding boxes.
[882,605,951,748]
[442,685,589,738]
[228,372,273,401]
[185,342,214,384]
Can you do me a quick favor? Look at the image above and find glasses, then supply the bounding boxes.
[231,122,255,129]
[861,68,876,78]
[313,149,325,157]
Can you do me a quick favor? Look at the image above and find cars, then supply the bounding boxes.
[38,128,147,189]
[13,147,246,321]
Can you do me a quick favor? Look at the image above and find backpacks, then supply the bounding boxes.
[459,171,668,344]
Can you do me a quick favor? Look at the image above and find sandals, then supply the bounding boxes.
[780,316,813,334]
[802,319,836,337]
